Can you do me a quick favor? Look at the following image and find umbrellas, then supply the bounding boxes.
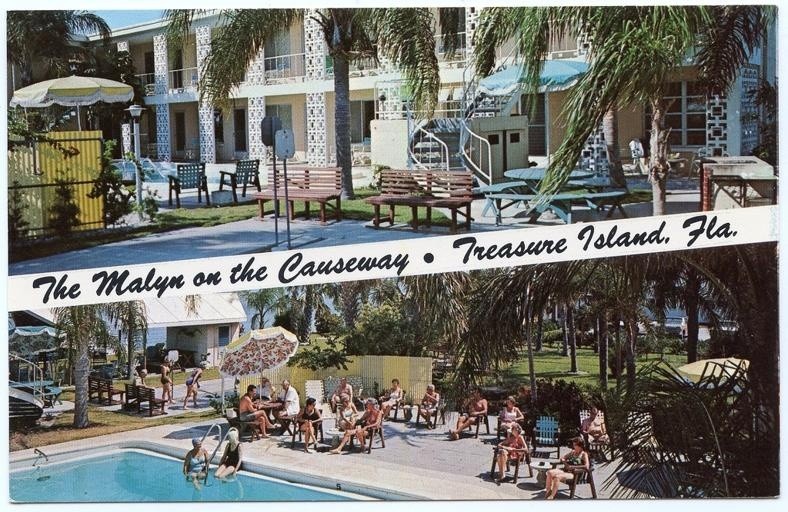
[218,326,300,401]
[678,356,750,415]
[479,58,593,169]
[8,75,134,131]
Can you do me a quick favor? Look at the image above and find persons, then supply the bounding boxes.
[582,407,612,446]
[496,423,529,483]
[133,369,162,408]
[159,358,177,405]
[183,360,207,410]
[500,395,525,438]
[240,376,440,454]
[544,437,589,500]
[183,437,210,480]
[449,387,487,440]
[214,431,242,480]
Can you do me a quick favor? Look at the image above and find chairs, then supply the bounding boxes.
[219,159,261,202]
[222,376,657,499]
[167,162,210,208]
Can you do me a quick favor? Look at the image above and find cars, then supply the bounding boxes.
[716,320,739,333]
[660,315,689,338]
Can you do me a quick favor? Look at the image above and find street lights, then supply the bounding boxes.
[125,103,147,222]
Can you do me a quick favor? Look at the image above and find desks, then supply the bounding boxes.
[504,168,595,212]
[11,381,53,387]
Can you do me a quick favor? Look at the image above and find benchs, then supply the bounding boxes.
[365,169,473,234]
[480,181,586,219]
[485,188,626,224]
[254,167,343,225]
[88,374,125,406]
[45,387,63,406]
[124,382,167,417]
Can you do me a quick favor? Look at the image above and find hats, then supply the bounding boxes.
[192,438,202,445]
